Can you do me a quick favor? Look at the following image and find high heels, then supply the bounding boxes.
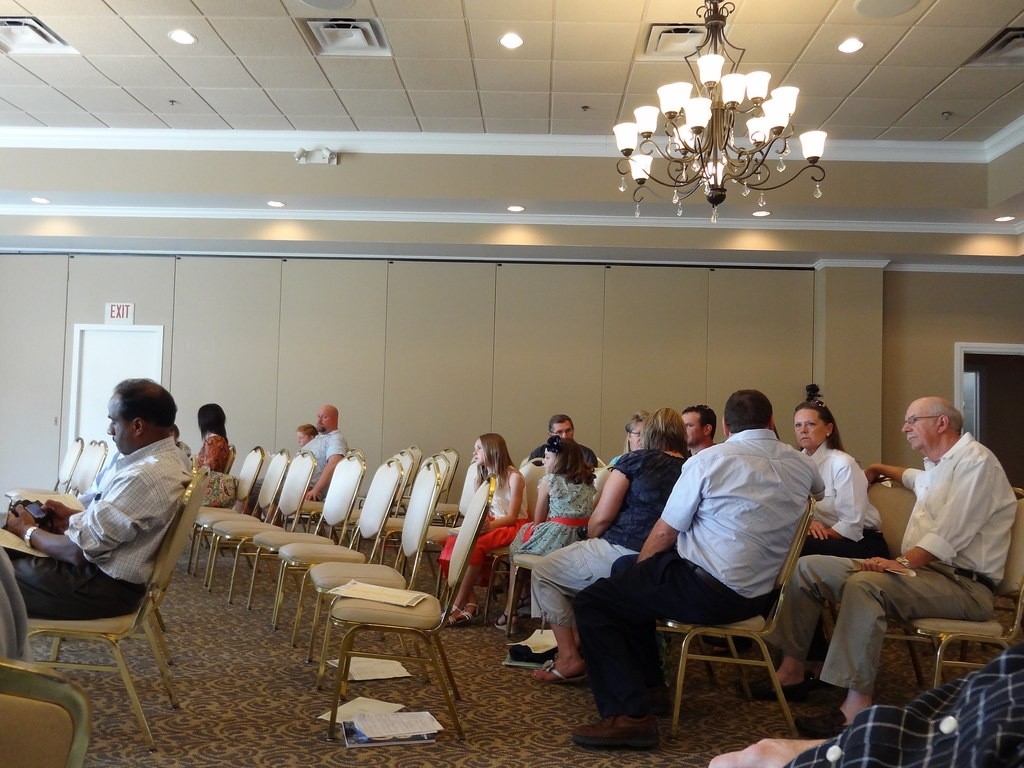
[516,597,532,615]
[494,611,519,634]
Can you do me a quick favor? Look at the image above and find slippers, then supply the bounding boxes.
[530,660,587,683]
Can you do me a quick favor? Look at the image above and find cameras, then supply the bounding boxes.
[10,500,53,526]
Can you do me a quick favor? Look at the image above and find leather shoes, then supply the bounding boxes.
[736,669,814,700]
[573,713,660,751]
[794,707,848,739]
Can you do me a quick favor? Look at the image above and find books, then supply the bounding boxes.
[342,719,436,748]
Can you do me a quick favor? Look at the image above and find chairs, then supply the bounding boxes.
[0,432,1024,768]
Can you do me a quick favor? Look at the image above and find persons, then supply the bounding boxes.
[681,404,717,456]
[438,433,532,626]
[531,408,688,683]
[297,423,319,447]
[232,405,349,525]
[527,410,656,469]
[173,403,230,474]
[571,390,825,749]
[708,644,1024,768]
[494,435,598,635]
[738,396,1018,737]
[793,399,890,559]
[0,378,194,620]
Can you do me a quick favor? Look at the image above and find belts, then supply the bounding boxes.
[954,566,997,593]
[683,558,754,607]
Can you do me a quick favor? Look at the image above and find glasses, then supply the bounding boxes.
[697,404,710,412]
[630,430,640,436]
[549,428,573,436]
[806,399,826,408]
[903,415,940,425]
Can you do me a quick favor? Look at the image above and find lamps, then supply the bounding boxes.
[612,0,830,221]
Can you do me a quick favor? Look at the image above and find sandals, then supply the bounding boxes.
[442,606,463,626]
[456,600,479,623]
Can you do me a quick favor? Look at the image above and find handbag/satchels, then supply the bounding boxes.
[182,472,238,508]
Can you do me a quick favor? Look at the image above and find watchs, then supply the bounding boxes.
[896,556,912,569]
[24,527,39,549]
